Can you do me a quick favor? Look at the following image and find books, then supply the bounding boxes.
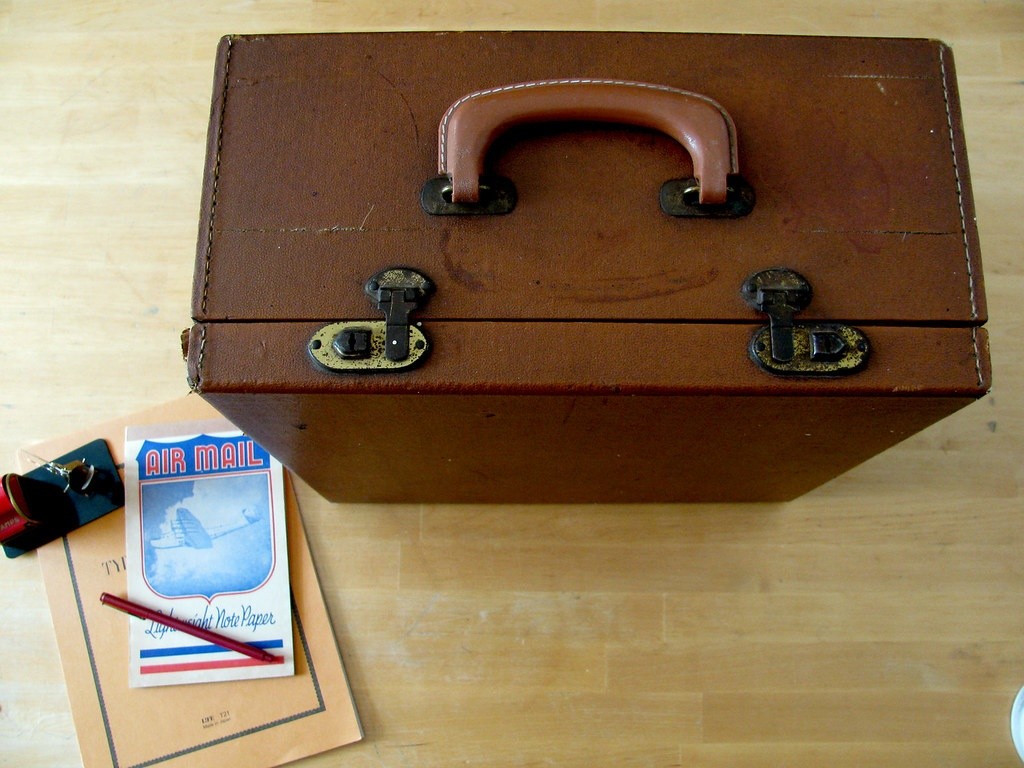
[17,393,363,768]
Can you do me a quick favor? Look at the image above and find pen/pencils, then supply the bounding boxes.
[100,589,275,663]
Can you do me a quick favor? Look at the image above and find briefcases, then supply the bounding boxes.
[183,31,991,505]
